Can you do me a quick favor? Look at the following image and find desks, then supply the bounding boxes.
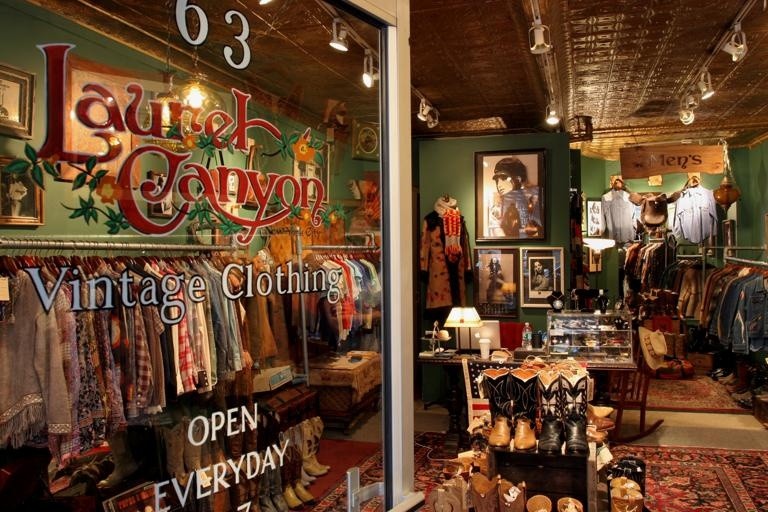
[415,342,640,448]
[307,344,387,439]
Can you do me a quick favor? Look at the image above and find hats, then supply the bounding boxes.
[492,157,527,182]
[636,324,668,371]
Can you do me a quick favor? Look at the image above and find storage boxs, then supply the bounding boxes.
[262,384,319,441]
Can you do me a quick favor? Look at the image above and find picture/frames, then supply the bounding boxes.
[472,146,551,246]
[56,56,142,191]
[1,64,38,141]
[695,233,715,258]
[3,154,45,227]
[518,242,567,311]
[471,244,524,321]
[585,244,602,274]
[145,170,174,219]
[586,199,602,237]
[721,218,737,257]
[198,119,333,228]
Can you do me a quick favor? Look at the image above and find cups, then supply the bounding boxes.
[479,339,496,360]
[532,333,542,348]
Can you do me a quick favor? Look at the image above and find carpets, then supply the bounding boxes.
[312,426,768,510]
[607,338,754,416]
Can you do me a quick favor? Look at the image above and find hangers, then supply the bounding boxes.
[676,248,768,280]
[1,230,386,284]
[612,175,626,191]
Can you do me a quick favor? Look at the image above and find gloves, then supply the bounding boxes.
[464,272,474,284]
[420,271,430,284]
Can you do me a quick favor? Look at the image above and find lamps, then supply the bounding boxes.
[443,305,484,357]
[669,15,756,130]
[713,135,741,214]
[318,9,443,133]
[528,8,563,128]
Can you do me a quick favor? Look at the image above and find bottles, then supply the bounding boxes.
[521,322,532,351]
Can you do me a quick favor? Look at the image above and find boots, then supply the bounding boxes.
[538,368,563,453]
[510,367,538,450]
[480,367,512,449]
[469,471,585,512]
[560,369,590,456]
[606,455,651,512]
[158,412,332,512]
[586,370,617,444]
[93,427,145,490]
[710,361,768,409]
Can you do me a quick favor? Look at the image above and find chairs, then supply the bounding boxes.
[596,314,673,449]
[496,321,531,348]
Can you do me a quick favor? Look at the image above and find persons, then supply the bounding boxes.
[491,159,544,239]
[487,256,504,305]
[532,261,552,291]
[418,194,472,311]
